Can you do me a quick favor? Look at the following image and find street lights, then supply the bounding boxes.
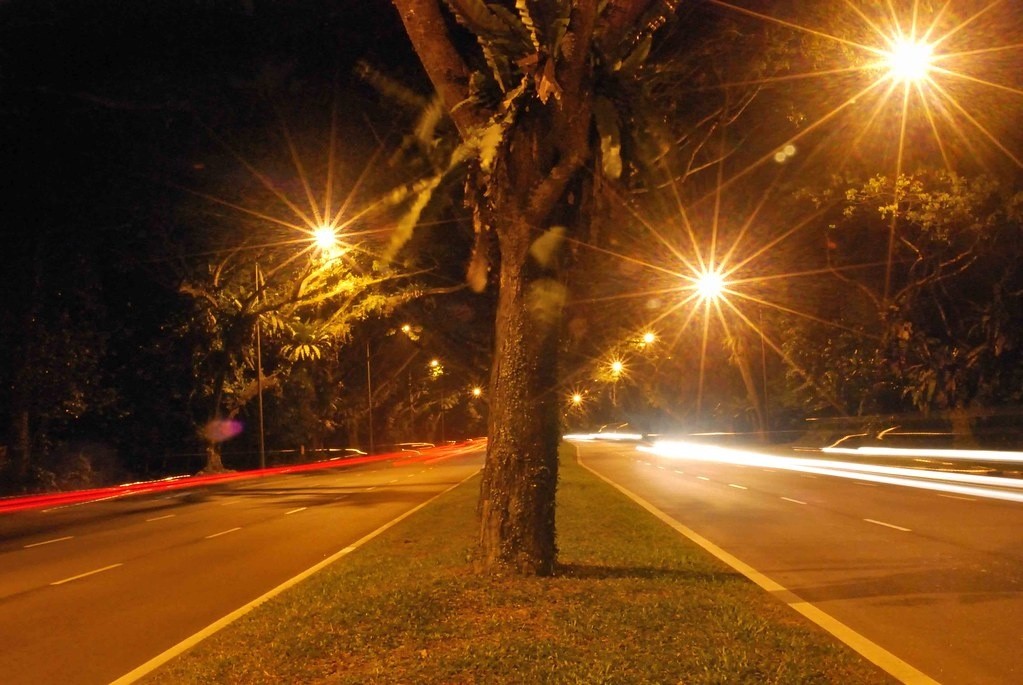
[366,322,412,454]
[252,226,342,470]
